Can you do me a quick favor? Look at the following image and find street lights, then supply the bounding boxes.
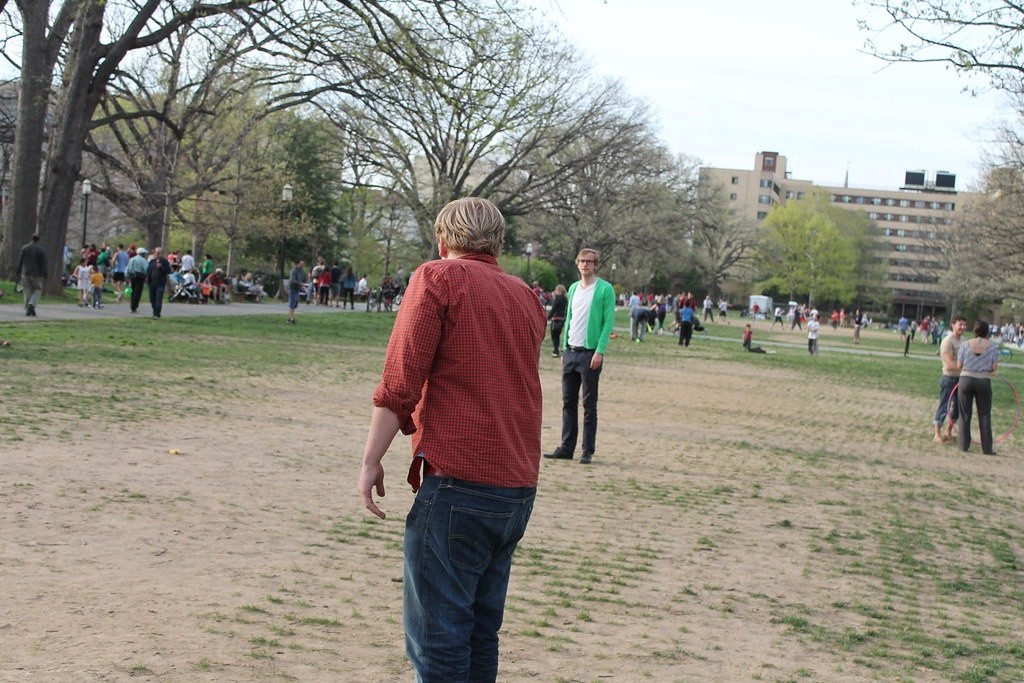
[82,179,94,247]
[526,243,533,273]
[612,262,617,284]
[275,182,294,300]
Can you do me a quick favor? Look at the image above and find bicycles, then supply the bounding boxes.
[993,339,1012,363]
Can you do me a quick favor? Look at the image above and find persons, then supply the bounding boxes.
[286,260,305,324]
[124,247,149,313]
[703,296,729,324]
[147,247,171,318]
[238,274,264,303]
[989,322,1024,347]
[619,290,698,347]
[167,249,227,303]
[934,316,967,446]
[743,324,752,353]
[529,279,550,304]
[359,197,547,683]
[548,284,568,357]
[957,321,998,454]
[64,242,137,309]
[16,234,48,315]
[543,249,616,463]
[383,264,414,286]
[770,303,818,332]
[807,315,820,355]
[830,308,869,344]
[753,302,759,314]
[899,314,944,346]
[306,259,368,311]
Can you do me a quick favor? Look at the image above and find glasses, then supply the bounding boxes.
[579,260,595,263]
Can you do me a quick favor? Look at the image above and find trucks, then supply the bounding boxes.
[749,295,774,314]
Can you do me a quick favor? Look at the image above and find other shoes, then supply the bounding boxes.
[26,310,31,315]
[286,318,297,323]
[553,353,559,356]
[944,434,956,441]
[579,451,591,462]
[27,305,36,316]
[118,292,124,299]
[544,446,573,459]
[983,451,996,455]
[934,436,943,444]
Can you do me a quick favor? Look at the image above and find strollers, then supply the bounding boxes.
[167,272,201,304]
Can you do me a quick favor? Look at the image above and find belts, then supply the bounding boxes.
[567,346,590,352]
[428,464,447,477]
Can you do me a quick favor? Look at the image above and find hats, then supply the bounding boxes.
[136,247,149,253]
